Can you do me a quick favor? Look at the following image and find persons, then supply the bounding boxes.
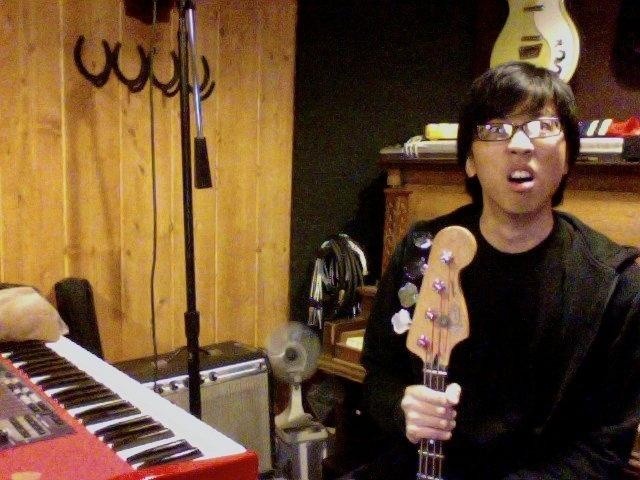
[356,59,639,477]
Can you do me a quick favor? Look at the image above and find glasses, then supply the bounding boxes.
[476,115,561,141]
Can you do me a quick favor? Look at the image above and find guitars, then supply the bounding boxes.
[400,223,480,479]
[489,0,581,87]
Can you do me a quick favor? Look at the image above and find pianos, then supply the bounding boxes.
[0,328,260,480]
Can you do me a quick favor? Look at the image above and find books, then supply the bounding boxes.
[403,116,639,162]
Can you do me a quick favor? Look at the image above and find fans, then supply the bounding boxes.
[265,324,330,443]
